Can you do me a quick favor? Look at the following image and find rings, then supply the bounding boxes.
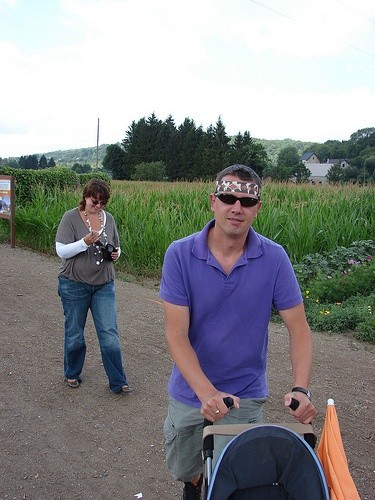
[215,410,219,415]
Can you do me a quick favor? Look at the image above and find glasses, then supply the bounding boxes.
[89,197,106,205]
[215,193,259,207]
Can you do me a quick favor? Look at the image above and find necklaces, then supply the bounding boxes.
[82,207,108,265]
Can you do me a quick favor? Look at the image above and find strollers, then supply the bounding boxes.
[200,397,361,500]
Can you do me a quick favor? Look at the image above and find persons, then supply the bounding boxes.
[53,178,134,395]
[160,163,317,500]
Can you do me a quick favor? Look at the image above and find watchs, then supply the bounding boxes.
[291,387,311,400]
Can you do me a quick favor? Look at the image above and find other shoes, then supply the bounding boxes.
[67,379,79,388]
[121,385,134,393]
[182,473,208,500]
